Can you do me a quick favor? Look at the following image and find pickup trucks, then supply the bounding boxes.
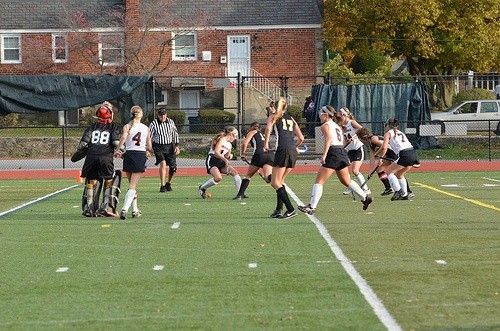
[429,100,500,136]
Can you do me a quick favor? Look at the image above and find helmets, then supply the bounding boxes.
[97,107,111,119]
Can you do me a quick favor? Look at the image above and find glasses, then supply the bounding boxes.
[385,123,387,127]
[319,113,328,117]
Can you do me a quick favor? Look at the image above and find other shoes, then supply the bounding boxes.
[165,182,172,191]
[96,208,117,217]
[82,209,95,217]
[132,211,142,217]
[160,186,165,192]
[120,210,128,220]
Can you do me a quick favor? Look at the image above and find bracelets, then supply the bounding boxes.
[147,149,150,151]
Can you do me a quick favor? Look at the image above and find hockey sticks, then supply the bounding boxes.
[351,160,384,200]
[266,144,308,154]
[374,155,421,168]
[243,158,272,183]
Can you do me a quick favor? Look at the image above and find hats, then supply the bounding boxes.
[158,108,167,114]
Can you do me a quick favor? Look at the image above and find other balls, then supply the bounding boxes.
[207,194,212,198]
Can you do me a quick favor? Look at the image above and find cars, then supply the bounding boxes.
[495,84,500,99]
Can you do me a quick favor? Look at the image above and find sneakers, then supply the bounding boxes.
[233,195,246,201]
[381,188,394,196]
[199,185,207,199]
[360,196,373,210]
[363,188,371,194]
[298,204,316,215]
[279,209,298,219]
[343,187,352,195]
[271,209,282,218]
[391,189,408,201]
[408,190,415,198]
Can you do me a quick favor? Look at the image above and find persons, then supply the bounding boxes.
[263,101,305,220]
[232,122,275,200]
[199,127,249,199]
[298,105,372,214]
[470,104,477,112]
[304,96,310,121]
[357,117,418,200]
[147,107,181,192]
[78,101,151,221]
[338,107,371,194]
